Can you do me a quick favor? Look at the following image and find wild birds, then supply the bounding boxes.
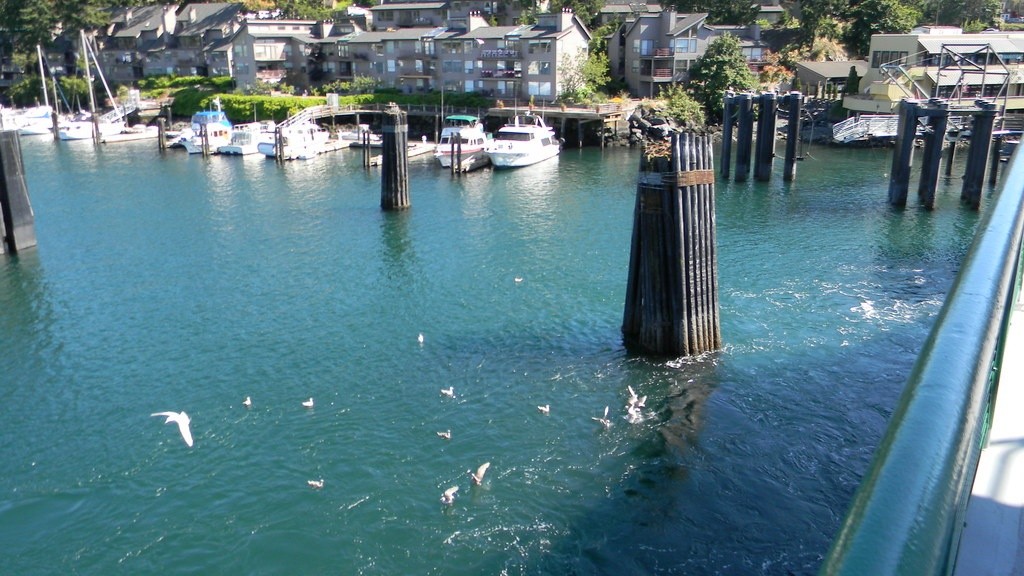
[515,277,523,282]
[466,462,490,485]
[150,411,194,448]
[441,387,454,397]
[622,384,656,424]
[591,405,610,426]
[437,430,450,439]
[301,397,314,407]
[537,404,550,414]
[307,479,324,489]
[439,486,459,505]
[243,396,251,406]
[418,333,424,344]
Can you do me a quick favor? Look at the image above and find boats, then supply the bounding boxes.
[216,120,278,157]
[182,109,235,156]
[257,122,349,162]
[0,101,97,142]
[96,122,161,144]
[435,113,493,173]
[483,110,564,169]
